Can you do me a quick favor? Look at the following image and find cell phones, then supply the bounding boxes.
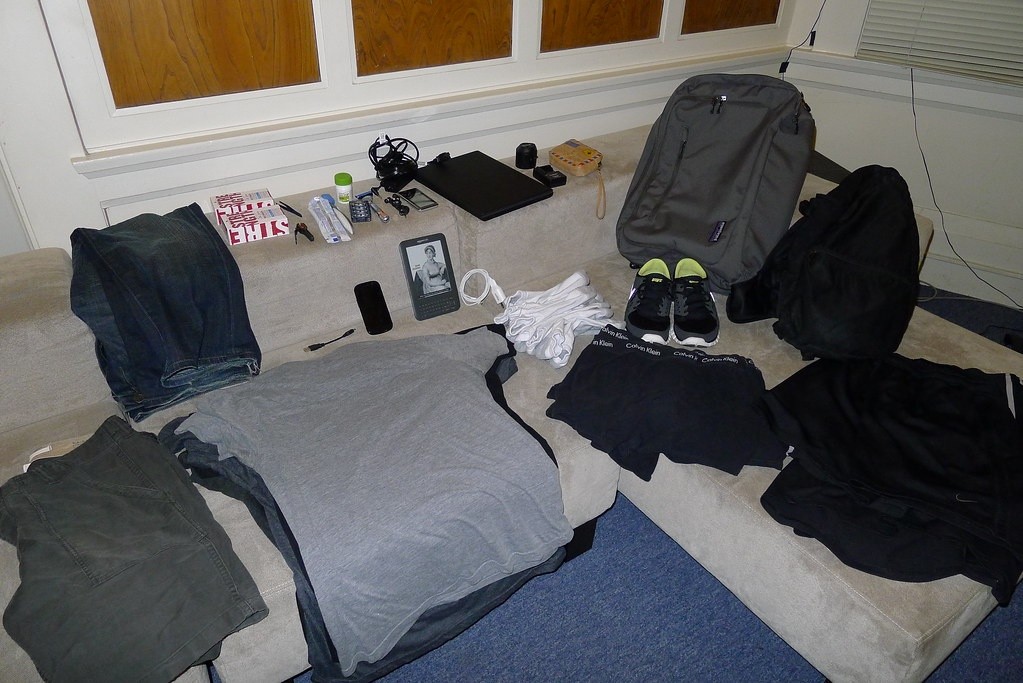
[354,281,392,336]
[398,188,439,211]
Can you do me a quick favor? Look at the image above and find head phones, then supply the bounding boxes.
[374,149,418,194]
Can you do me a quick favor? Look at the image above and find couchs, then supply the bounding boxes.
[0,125,1023,683]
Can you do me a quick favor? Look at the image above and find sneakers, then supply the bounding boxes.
[624,258,673,346]
[672,258,720,348]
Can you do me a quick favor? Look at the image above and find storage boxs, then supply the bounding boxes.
[220,205,289,244]
[211,188,275,225]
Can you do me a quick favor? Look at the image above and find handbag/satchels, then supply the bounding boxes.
[727,164,919,360]
[549,139,606,220]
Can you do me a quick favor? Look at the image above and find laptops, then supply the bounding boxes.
[415,150,554,221]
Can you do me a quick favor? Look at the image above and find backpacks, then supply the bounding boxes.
[616,74,853,295]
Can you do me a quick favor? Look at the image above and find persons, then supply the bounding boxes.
[421,245,449,294]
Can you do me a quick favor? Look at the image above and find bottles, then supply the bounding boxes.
[335,173,353,204]
